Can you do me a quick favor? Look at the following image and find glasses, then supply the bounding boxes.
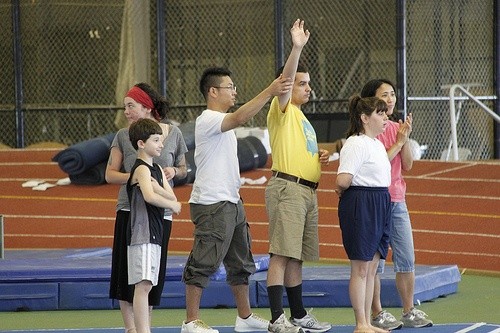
[208,85,237,93]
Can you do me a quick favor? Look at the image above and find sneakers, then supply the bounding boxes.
[267,311,305,333]
[288,307,332,333]
[234,313,270,332]
[370,309,404,330]
[400,307,433,328]
[180,319,219,333]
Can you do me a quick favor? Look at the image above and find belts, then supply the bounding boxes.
[271,170,319,190]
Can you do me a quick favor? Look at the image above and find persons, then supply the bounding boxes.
[182,67,294,333]
[266,18,333,333]
[126,118,182,333]
[105,82,188,333]
[359,79,434,331]
[334,96,391,333]
